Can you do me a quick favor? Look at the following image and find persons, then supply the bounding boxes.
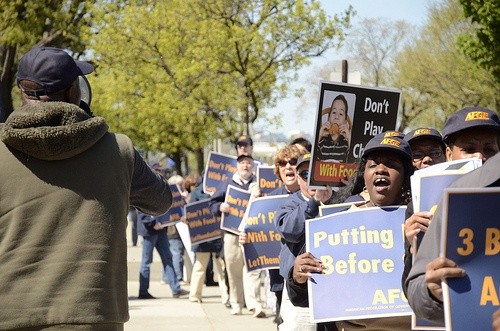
[129,135,267,318]
[315,95,352,163]
[240,107,500,331]
[0,46,173,331]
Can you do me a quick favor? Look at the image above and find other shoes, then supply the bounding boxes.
[231,306,241,315]
[138,293,155,298]
[253,308,266,318]
[173,289,189,299]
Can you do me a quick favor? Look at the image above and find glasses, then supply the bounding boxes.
[279,158,297,166]
[299,169,308,179]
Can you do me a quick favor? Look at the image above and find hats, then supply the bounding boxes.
[296,154,311,168]
[234,135,252,146]
[441,106,499,138]
[16,46,95,96]
[375,130,405,138]
[236,152,253,161]
[363,138,413,169]
[403,127,441,144]
